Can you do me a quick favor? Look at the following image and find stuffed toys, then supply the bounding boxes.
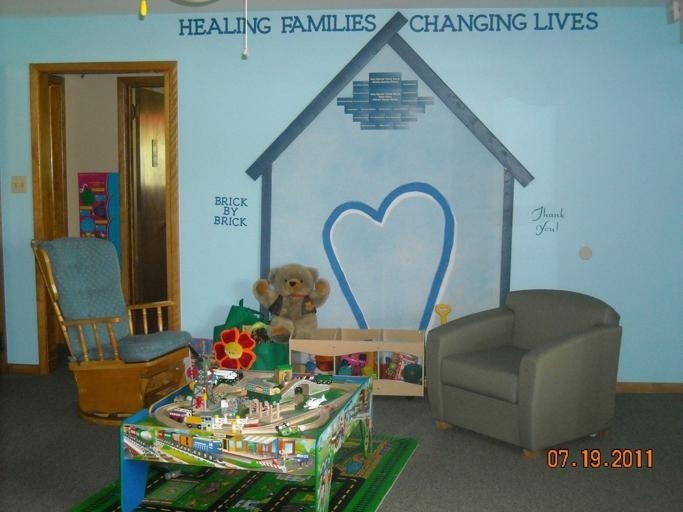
[253,264,330,343]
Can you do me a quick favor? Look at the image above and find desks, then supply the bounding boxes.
[119,373,373,511]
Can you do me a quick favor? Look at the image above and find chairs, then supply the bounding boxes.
[31,236,192,427]
[424,290,623,459]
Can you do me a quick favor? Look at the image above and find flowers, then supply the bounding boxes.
[214,320,267,371]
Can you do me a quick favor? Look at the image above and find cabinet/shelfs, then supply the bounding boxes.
[289,325,425,397]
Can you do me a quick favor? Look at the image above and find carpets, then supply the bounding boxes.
[66,431,424,511]
